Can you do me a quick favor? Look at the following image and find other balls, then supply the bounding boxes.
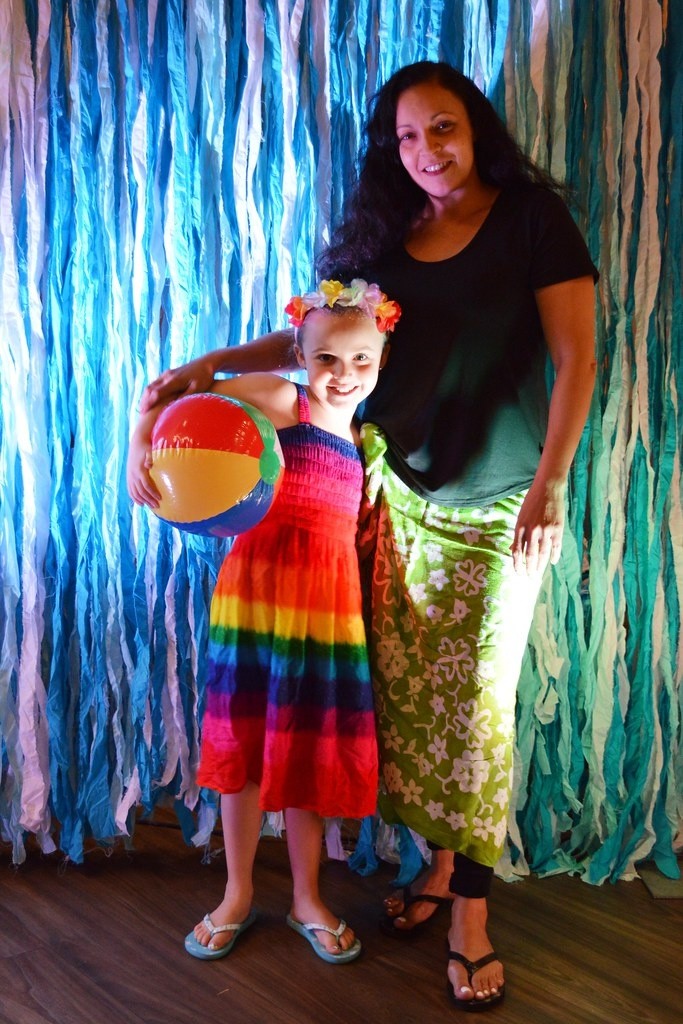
[144,391,287,539]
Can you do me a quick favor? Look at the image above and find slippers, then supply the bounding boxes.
[381,874,455,938]
[184,907,257,959]
[445,935,507,1011]
[287,913,361,963]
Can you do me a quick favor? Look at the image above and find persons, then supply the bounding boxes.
[138,60,600,1009]
[127,265,401,964]
